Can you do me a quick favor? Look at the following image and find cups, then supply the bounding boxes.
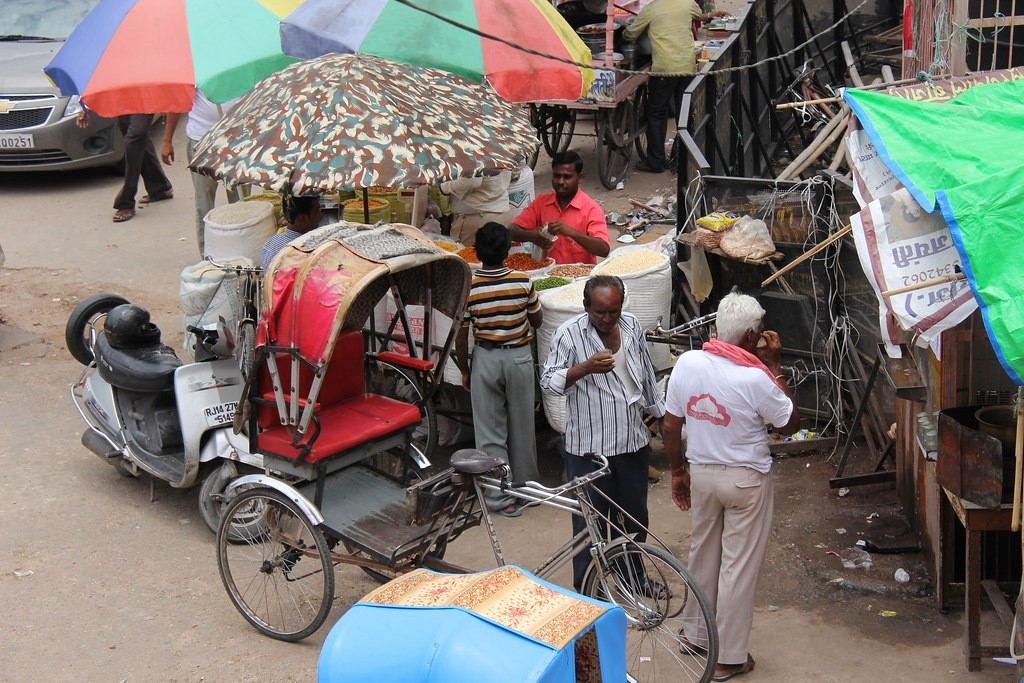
[598,350,612,363]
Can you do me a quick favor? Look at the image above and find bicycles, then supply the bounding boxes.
[198,255,265,381]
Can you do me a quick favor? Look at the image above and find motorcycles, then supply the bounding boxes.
[63,291,288,545]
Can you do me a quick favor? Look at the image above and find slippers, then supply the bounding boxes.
[712,652,755,681]
[497,503,521,517]
[678,625,703,654]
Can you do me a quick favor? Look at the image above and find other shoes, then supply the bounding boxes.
[139,187,173,203]
[638,579,672,599]
[113,209,135,221]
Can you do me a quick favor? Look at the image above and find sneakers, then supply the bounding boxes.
[636,160,665,173]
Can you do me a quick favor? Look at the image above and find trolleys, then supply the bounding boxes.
[518,64,655,192]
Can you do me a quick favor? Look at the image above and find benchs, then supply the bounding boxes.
[249,327,439,516]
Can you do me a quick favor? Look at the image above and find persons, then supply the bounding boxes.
[75,93,174,222]
[661,294,802,682]
[258,181,323,296]
[454,220,545,519]
[538,273,673,606]
[160,76,260,264]
[506,150,611,267]
[619,0,704,172]
[439,165,512,250]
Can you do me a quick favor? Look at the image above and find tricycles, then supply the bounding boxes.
[357,283,743,472]
[213,223,720,683]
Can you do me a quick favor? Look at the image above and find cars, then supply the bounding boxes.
[1,0,150,178]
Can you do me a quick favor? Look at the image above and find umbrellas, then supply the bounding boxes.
[43,0,307,118]
[183,50,544,377]
[277,0,596,106]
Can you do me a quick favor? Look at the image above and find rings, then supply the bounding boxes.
[773,338,778,342]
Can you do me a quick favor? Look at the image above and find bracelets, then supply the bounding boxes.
[774,373,786,381]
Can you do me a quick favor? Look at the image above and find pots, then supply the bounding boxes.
[975,404,1017,456]
[627,15,652,55]
[620,45,639,72]
[577,22,620,38]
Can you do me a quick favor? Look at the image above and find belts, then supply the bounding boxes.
[474,340,528,350]
